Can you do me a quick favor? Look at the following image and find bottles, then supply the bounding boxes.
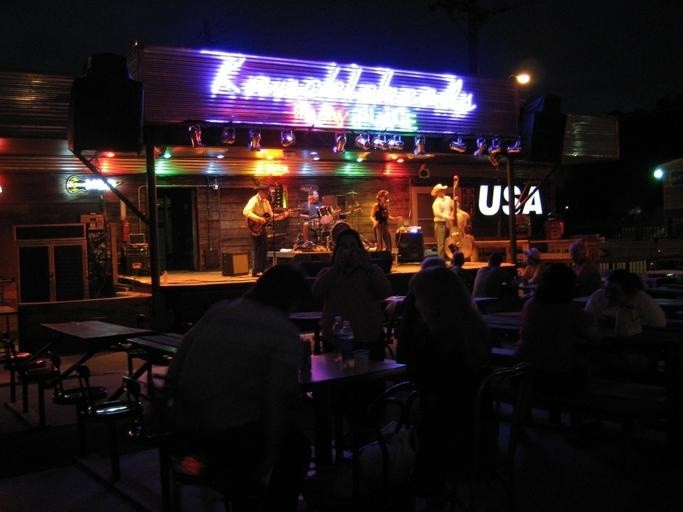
[331,315,344,368]
[340,321,356,371]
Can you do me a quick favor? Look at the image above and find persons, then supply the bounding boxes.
[430,183,458,257]
[369,189,402,252]
[446,202,473,262]
[159,228,666,511]
[298,190,325,249]
[242,180,290,278]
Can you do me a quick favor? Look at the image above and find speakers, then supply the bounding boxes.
[70,56,143,152]
[223,251,249,276]
[365,251,392,273]
[518,98,567,160]
[396,233,424,262]
[288,254,329,277]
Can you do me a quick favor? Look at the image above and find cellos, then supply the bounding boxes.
[444,176,475,259]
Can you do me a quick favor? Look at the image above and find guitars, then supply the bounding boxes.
[247,208,305,236]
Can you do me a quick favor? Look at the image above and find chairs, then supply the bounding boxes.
[1,317,143,490]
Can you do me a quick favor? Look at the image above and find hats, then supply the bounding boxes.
[431,184,448,197]
[422,257,453,269]
[525,248,540,259]
[454,251,464,263]
[255,182,271,189]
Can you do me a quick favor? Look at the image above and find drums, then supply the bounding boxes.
[311,219,321,230]
[317,206,333,224]
[326,220,353,246]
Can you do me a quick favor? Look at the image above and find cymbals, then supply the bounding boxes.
[341,208,361,217]
[336,192,360,196]
[300,184,319,192]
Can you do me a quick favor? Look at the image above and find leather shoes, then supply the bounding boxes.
[249,270,264,276]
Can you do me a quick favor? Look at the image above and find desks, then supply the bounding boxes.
[40,288,683,512]
